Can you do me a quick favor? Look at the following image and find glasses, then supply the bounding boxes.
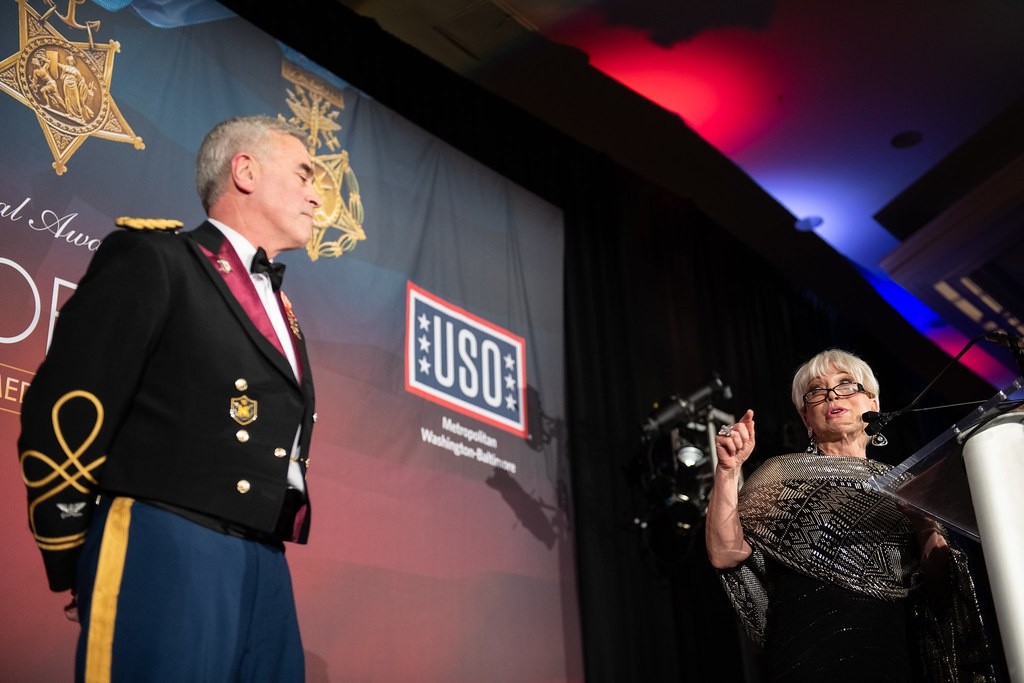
[802,381,872,405]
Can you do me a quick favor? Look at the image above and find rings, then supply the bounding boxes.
[719,425,733,437]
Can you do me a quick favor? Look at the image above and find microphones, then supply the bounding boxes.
[861,329,1024,436]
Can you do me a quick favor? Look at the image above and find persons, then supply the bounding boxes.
[18,116,321,683]
[707,349,998,683]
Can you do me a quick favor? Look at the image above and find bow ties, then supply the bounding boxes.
[251,245,288,295]
